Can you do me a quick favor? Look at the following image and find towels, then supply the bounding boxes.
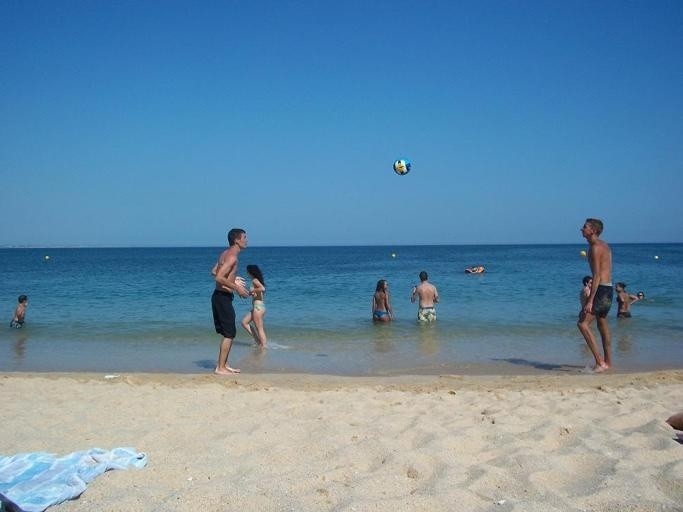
[2,446,154,512]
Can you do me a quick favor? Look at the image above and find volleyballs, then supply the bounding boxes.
[393,158,411,175]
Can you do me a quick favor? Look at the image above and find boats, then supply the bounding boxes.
[465,267,483,273]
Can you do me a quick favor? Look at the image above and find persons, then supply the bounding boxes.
[637,291,645,301]
[211,229,248,375]
[665,411,683,441]
[371,280,396,321]
[615,282,638,319]
[240,264,267,348]
[411,271,439,322]
[579,276,594,310]
[10,294,28,329]
[476,266,484,273]
[576,218,612,372]
